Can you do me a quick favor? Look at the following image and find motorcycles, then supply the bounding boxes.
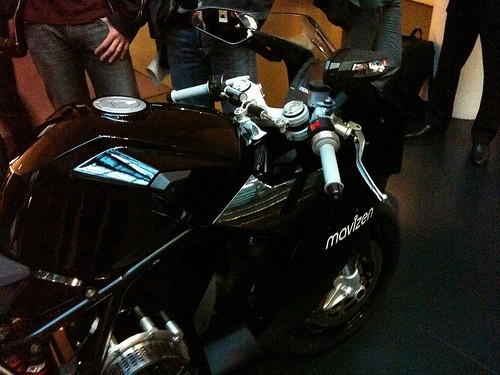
[0,6,402,375]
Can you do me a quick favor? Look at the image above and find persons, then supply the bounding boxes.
[0,0,259,174]
[313,0,402,91]
[404,0,500,167]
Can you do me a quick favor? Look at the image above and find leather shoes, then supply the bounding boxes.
[402,120,446,141]
[468,141,489,168]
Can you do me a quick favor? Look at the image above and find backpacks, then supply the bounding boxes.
[391,27,434,113]
